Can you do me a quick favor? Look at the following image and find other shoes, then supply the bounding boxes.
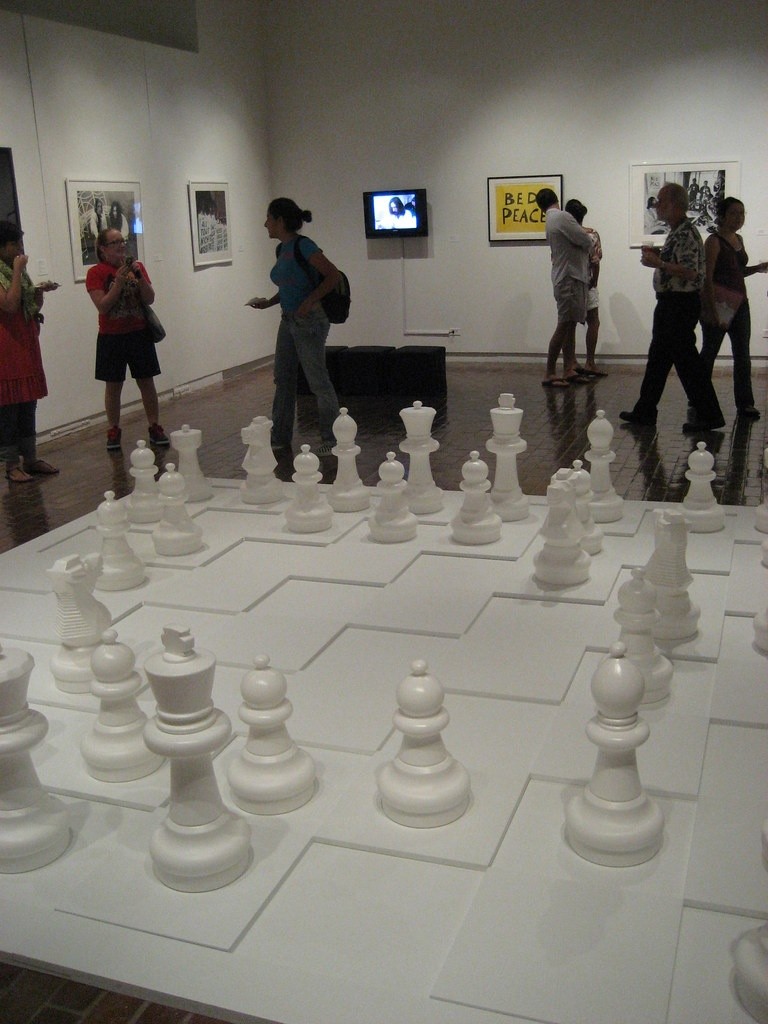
[682,417,726,434]
[106,425,122,448]
[271,438,284,449]
[736,405,759,416]
[148,422,170,444]
[317,443,336,456]
[619,410,657,425]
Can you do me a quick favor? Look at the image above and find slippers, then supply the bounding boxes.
[541,377,572,387]
[565,371,590,384]
[575,367,608,376]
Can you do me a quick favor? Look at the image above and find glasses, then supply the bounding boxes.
[106,238,128,246]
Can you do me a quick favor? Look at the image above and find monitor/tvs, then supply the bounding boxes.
[363,188,428,239]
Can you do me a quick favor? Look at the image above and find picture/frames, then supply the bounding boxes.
[486,174,564,242]
[189,179,233,267]
[0,147,22,230]
[65,179,145,285]
[629,160,742,249]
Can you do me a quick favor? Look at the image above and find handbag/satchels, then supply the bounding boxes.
[138,293,166,343]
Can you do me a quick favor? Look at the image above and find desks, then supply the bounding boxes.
[0,476,768,1024]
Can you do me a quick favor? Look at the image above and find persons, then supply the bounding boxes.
[535,188,608,385]
[378,197,416,228]
[245,198,339,456]
[619,183,726,432]
[646,170,725,230]
[0,221,60,483]
[90,199,129,241]
[699,197,768,415]
[86,228,169,449]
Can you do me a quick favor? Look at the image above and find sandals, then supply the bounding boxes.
[23,458,60,474]
[5,467,33,482]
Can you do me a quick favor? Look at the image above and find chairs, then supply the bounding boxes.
[298,346,446,396]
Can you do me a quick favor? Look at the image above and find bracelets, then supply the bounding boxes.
[660,261,665,269]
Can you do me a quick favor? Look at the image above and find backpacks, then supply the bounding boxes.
[275,234,350,325]
